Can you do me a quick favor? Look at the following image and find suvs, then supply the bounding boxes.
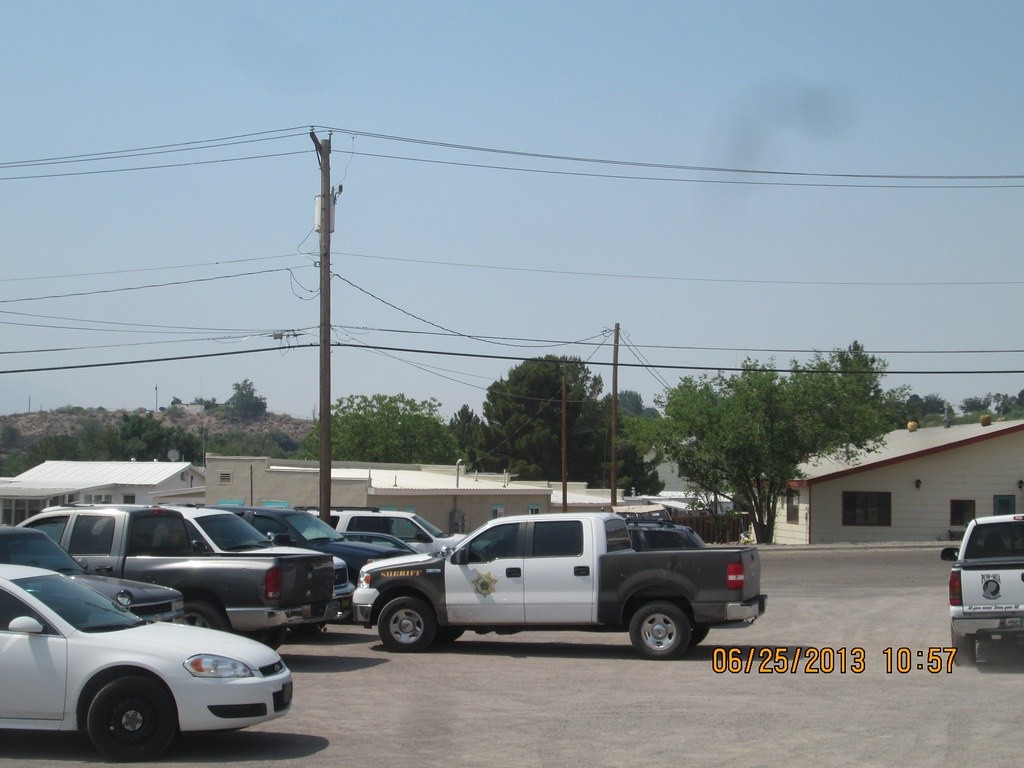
[292,503,468,554]
[624,518,706,550]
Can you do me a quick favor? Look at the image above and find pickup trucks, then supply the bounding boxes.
[352,512,768,660]
[16,507,335,651]
[941,514,1024,667]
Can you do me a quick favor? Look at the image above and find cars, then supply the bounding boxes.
[162,502,356,635]
[340,531,421,555]
[0,563,292,762]
[214,505,413,587]
[0,524,186,624]
